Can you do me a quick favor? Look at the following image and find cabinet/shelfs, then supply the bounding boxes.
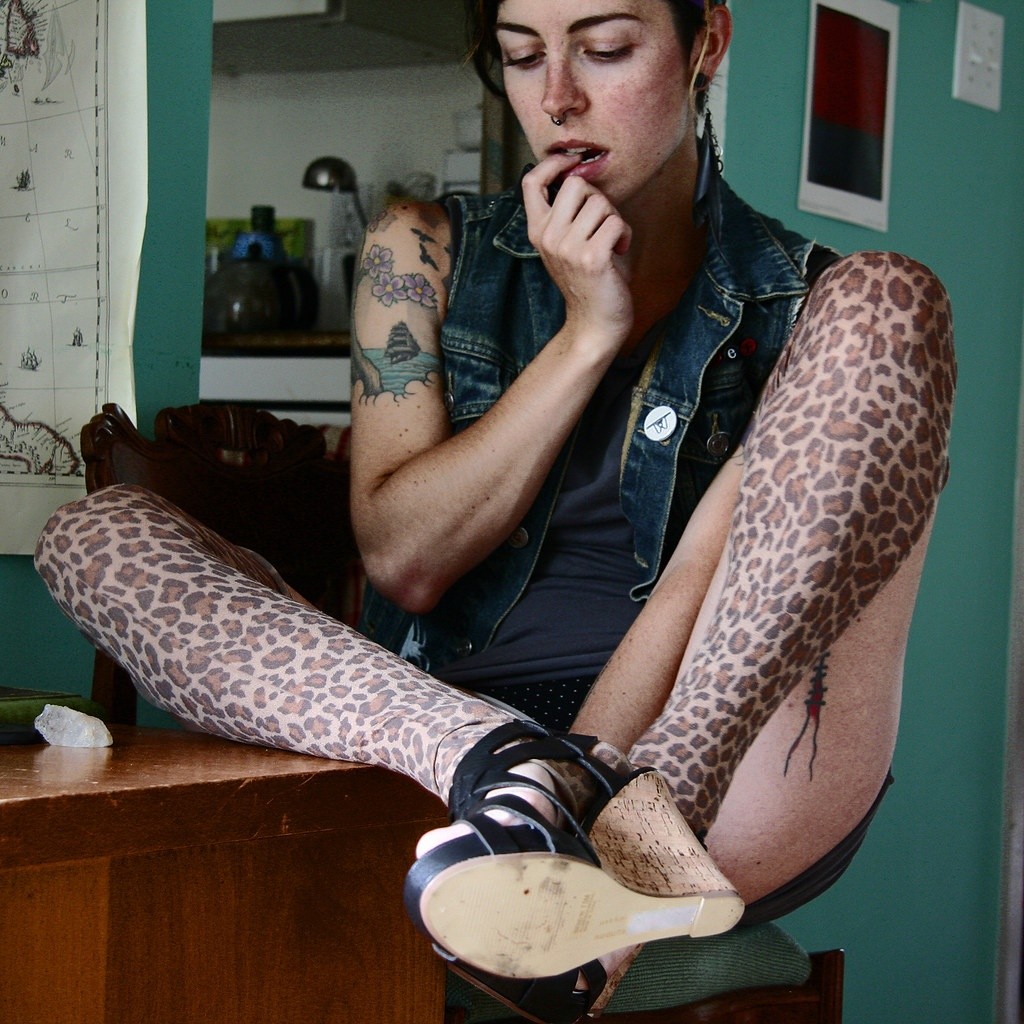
[0,715,447,1024]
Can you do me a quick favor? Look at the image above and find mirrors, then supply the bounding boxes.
[201,1,489,359]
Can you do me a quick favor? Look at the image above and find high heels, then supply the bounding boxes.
[404,720,746,978]
[430,940,647,1024]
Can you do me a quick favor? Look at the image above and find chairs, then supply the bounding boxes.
[74,399,843,1023]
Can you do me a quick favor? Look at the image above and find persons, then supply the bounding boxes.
[33,0,958,1024]
